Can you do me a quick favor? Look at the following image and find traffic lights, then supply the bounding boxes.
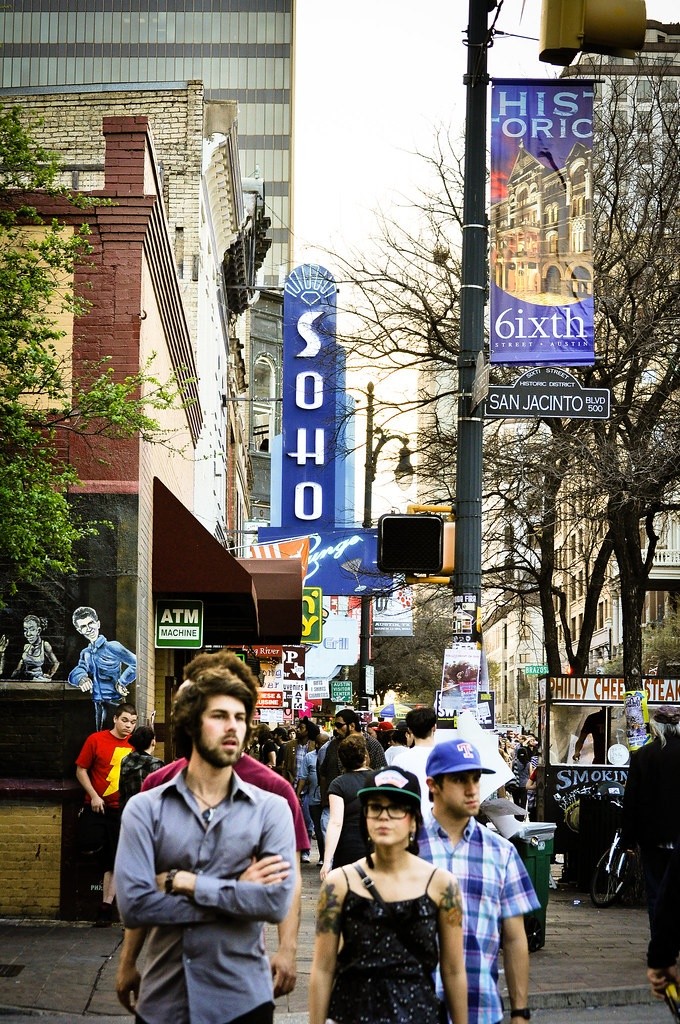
[539,0,646,67]
[377,512,445,575]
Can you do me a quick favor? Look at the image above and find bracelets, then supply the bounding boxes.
[165,869,178,893]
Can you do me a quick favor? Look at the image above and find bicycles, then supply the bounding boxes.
[589,796,636,909]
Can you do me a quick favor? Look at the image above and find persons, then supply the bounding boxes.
[572,706,611,765]
[119,726,164,818]
[647,848,680,1001]
[75,702,138,928]
[242,709,436,865]
[491,728,557,821]
[114,644,310,1015]
[621,706,680,932]
[414,738,542,1024]
[307,767,469,1024]
[394,706,442,827]
[114,671,299,1024]
[317,734,375,951]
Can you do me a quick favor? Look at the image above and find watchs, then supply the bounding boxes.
[510,1008,530,1019]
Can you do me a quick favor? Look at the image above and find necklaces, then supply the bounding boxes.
[189,788,228,824]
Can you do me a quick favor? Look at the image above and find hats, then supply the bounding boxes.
[372,722,399,732]
[356,766,422,806]
[426,740,496,776]
[269,727,286,735]
[653,705,680,725]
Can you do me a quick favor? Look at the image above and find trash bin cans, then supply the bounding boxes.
[483,820,558,952]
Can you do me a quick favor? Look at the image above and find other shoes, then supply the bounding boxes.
[316,860,324,866]
[312,832,317,839]
[300,853,310,863]
[94,904,113,926]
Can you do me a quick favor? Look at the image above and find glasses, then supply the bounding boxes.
[332,722,347,730]
[363,803,411,820]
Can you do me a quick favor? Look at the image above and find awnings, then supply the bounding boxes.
[149,474,262,641]
[231,555,306,645]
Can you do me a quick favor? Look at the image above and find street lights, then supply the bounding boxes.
[358,379,415,731]
